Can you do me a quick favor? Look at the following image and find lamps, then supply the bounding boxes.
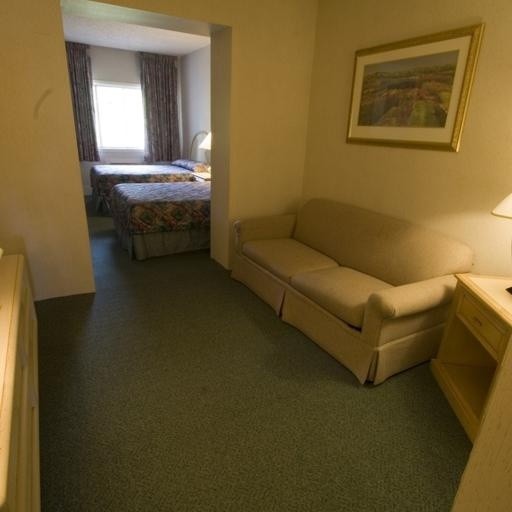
[199,131,211,167]
[491,194,510,297]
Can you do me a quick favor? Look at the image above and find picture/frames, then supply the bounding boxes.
[344,22,486,153]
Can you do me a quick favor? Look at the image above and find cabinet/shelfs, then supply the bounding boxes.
[429,269,512,441]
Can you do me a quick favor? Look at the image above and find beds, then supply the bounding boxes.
[89,158,214,261]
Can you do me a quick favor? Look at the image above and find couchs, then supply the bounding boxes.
[230,197,475,388]
